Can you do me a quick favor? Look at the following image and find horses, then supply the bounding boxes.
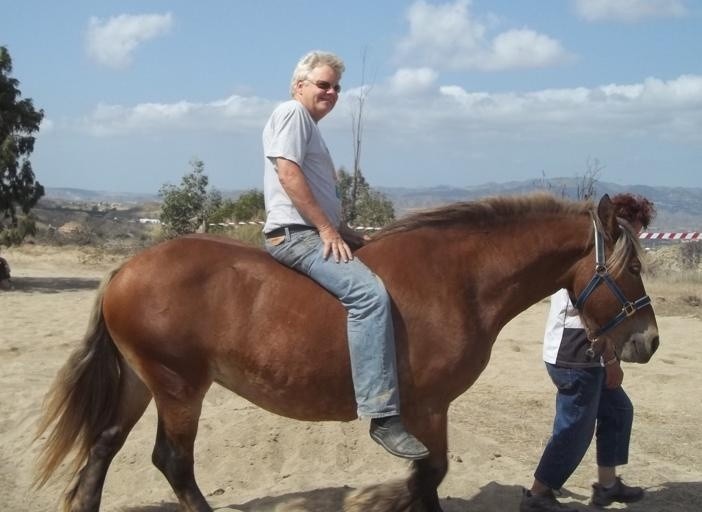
[14,189,660,512]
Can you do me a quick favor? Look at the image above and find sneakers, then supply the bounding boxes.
[591,477,643,505]
[519,489,576,512]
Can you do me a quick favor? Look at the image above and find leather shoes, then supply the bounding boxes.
[369,419,430,460]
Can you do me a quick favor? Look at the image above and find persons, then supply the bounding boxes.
[262,49,430,460]
[519,194,658,512]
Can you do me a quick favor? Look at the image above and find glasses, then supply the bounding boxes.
[306,79,340,93]
